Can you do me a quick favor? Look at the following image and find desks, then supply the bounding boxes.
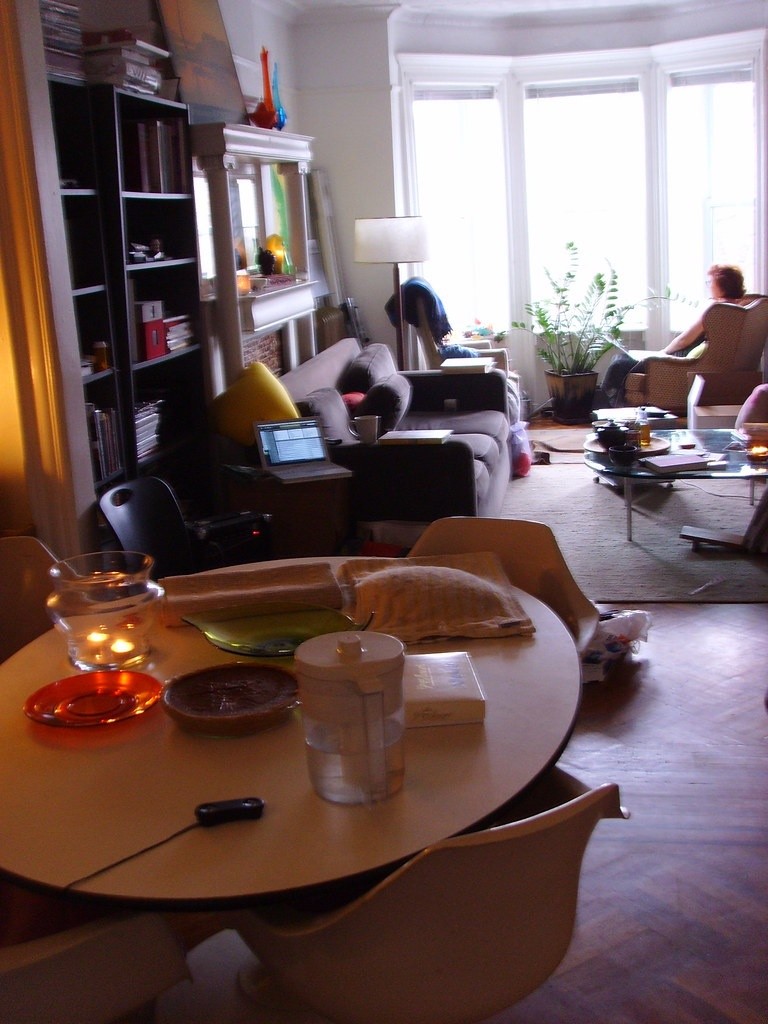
[587,430,768,542]
[199,276,323,380]
[2,555,584,904]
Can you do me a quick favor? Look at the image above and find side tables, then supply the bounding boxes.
[229,454,352,555]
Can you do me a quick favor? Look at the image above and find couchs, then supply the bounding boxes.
[218,337,514,520]
[384,276,523,391]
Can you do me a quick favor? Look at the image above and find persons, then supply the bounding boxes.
[596,265,749,407]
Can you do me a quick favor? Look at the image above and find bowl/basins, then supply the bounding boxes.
[594,419,630,449]
[162,661,300,737]
[608,445,637,466]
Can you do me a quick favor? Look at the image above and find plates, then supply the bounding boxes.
[22,671,164,729]
[584,436,671,454]
[181,601,374,658]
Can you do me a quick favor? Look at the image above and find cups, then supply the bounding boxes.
[93,341,109,372]
[44,550,166,672]
[347,415,378,443]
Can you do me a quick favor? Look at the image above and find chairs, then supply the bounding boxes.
[0,469,632,1024]
[618,298,768,415]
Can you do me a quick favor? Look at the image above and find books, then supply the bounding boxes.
[134,299,194,362]
[86,400,123,482]
[39,0,169,97]
[641,453,728,474]
[133,397,165,461]
[123,116,192,193]
[725,419,768,453]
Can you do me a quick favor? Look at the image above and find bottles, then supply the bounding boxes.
[634,407,651,446]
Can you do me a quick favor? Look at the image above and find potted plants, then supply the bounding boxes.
[511,243,696,425]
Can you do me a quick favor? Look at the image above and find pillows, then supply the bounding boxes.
[294,386,355,440]
[344,345,397,394]
[352,373,411,434]
[222,361,300,447]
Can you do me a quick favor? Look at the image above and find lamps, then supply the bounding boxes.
[354,215,433,370]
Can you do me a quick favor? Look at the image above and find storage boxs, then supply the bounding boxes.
[686,370,763,431]
[594,404,677,441]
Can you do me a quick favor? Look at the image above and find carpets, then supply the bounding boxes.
[486,427,768,604]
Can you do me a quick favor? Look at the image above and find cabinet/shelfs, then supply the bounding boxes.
[54,78,215,506]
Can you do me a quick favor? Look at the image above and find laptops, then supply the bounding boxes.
[252,415,352,484]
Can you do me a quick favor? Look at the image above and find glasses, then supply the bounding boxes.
[706,280,715,287]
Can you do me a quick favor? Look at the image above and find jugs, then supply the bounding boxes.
[293,630,407,805]
